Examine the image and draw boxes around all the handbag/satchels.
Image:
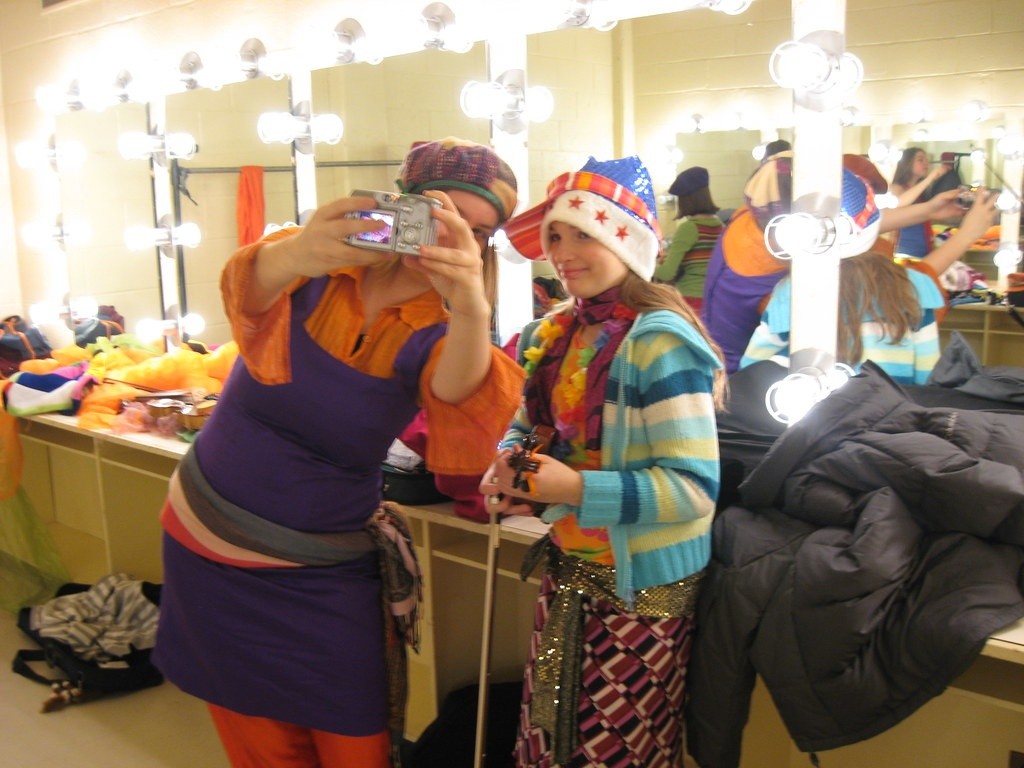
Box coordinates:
[12,581,165,714]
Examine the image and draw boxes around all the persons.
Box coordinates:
[479,153,727,768]
[156,134,526,768]
[652,141,1002,385]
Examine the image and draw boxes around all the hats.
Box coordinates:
[744,154,887,260]
[395,136,518,229]
[668,166,708,195]
[502,155,660,284]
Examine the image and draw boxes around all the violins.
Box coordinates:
[505,422,556,525]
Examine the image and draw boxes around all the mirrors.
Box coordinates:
[52,0,1023,372]
[676,114,1023,285]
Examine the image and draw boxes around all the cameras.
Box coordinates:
[344,189,444,254]
[954,183,1002,210]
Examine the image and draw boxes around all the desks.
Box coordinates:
[0,381,1024,768]
[938,302,1023,372]
